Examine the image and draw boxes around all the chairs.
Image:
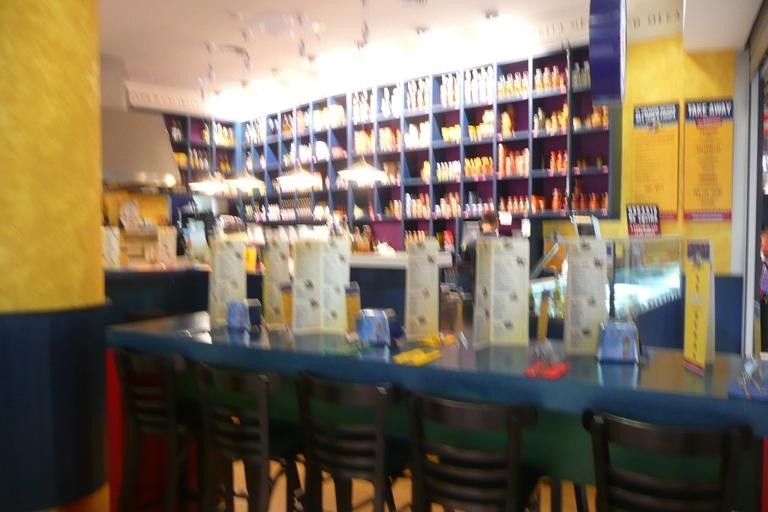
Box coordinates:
[117,345,753,511]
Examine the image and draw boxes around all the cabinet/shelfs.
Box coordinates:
[162,41,620,252]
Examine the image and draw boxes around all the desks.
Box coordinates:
[105,309,768,511]
